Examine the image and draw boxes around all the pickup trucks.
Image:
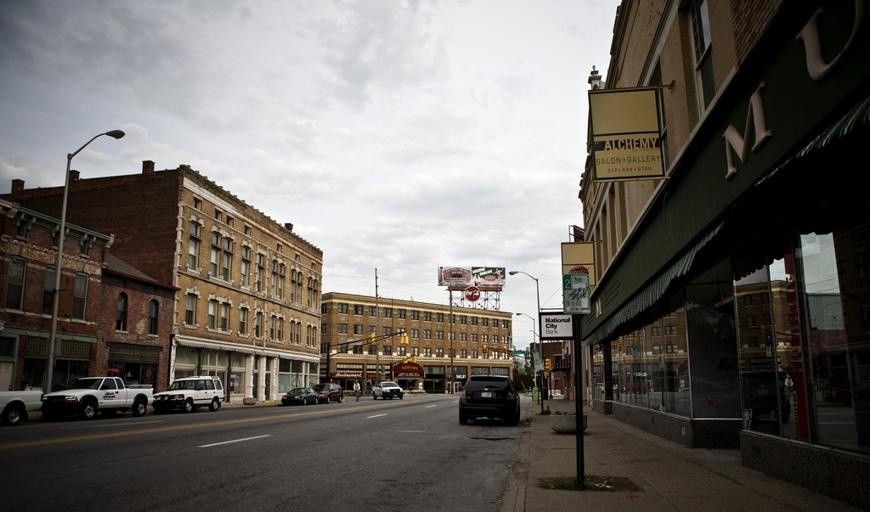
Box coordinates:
[0,376,155,425]
[372,381,403,400]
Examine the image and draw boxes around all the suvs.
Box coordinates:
[152,375,225,413]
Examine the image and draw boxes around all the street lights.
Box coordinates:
[509,271,543,413]
[46,130,125,394]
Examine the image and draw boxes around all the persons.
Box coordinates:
[353,379,360,396]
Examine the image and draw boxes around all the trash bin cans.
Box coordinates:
[532,387,539,400]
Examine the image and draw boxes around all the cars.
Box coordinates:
[459,374,519,427]
[281,383,343,406]
